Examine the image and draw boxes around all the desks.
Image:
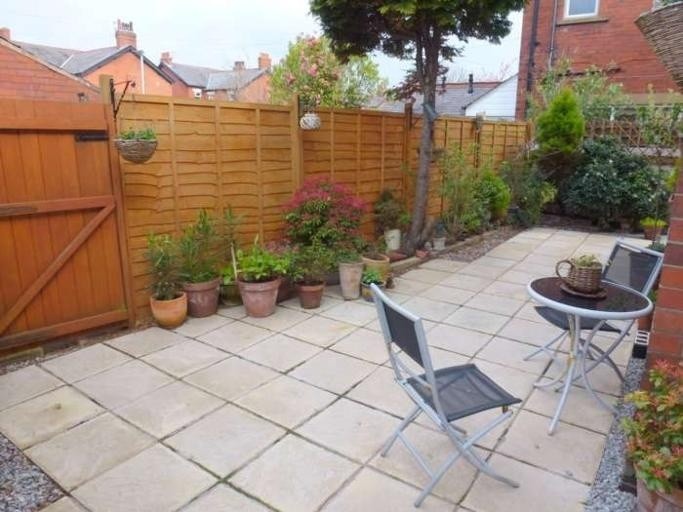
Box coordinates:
[523,272,652,435]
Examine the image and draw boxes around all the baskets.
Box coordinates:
[555,260,602,293]
[634,2,683,87]
[113,140,158,163]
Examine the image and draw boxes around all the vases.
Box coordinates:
[630,460,682,512]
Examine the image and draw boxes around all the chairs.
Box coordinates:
[366,281,524,507]
[523,238,663,392]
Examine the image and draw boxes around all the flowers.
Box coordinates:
[618,359,682,493]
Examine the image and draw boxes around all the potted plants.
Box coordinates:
[640,217,666,239]
[432,218,448,251]
[142,188,404,330]
[565,252,602,295]
[298,93,321,130]
[114,123,158,163]
